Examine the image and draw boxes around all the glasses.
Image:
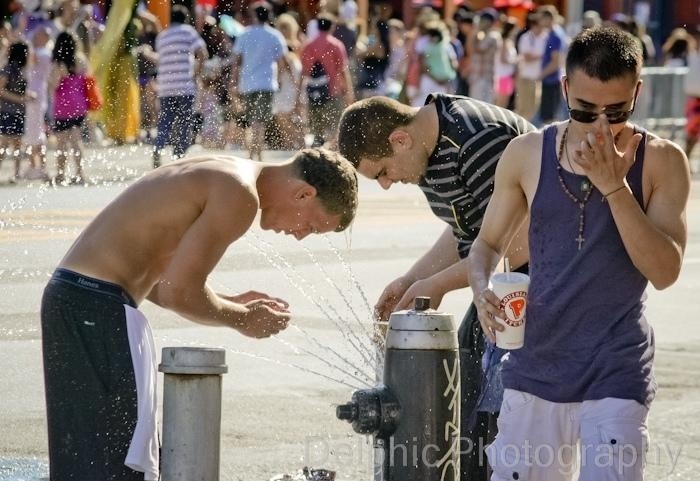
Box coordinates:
[564,81,640,125]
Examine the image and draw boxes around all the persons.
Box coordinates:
[469,27,692,479]
[0,0,699,191]
[38,146,360,480]
[335,93,545,480]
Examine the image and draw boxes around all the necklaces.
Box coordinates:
[556,119,626,252]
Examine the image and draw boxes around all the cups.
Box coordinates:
[492,269,529,351]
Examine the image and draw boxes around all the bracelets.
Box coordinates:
[601,183,628,199]
